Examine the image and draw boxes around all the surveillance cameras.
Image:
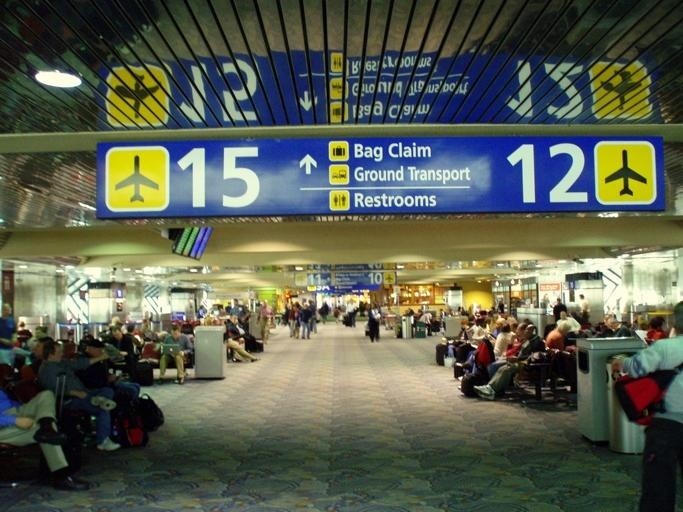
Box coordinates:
[573,259,583,264]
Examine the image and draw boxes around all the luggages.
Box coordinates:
[39,370,81,479]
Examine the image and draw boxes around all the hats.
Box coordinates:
[87,339,104,348]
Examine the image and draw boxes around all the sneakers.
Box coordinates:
[90,395,117,411]
[95,435,121,452]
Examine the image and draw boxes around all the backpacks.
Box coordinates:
[435,337,497,401]
[132,361,154,386]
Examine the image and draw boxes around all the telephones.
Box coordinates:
[575,335,644,454]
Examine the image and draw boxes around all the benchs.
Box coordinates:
[153,335,194,368]
[514,339,547,391]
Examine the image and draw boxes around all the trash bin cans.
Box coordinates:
[384,314,396,329]
[248,312,261,339]
[193,326,225,379]
[401,316,411,338]
[415,322,424,337]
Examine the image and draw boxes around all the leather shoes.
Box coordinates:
[54,474,89,490]
[32,428,67,446]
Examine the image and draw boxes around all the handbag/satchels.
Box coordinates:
[111,391,165,448]
[614,369,677,422]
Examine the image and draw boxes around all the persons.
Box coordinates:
[0,298,274,490]
[334,299,383,342]
[609,301,683,511]
[281,298,328,339]
[441,294,638,400]
[385,308,433,336]
[644,317,666,343]
